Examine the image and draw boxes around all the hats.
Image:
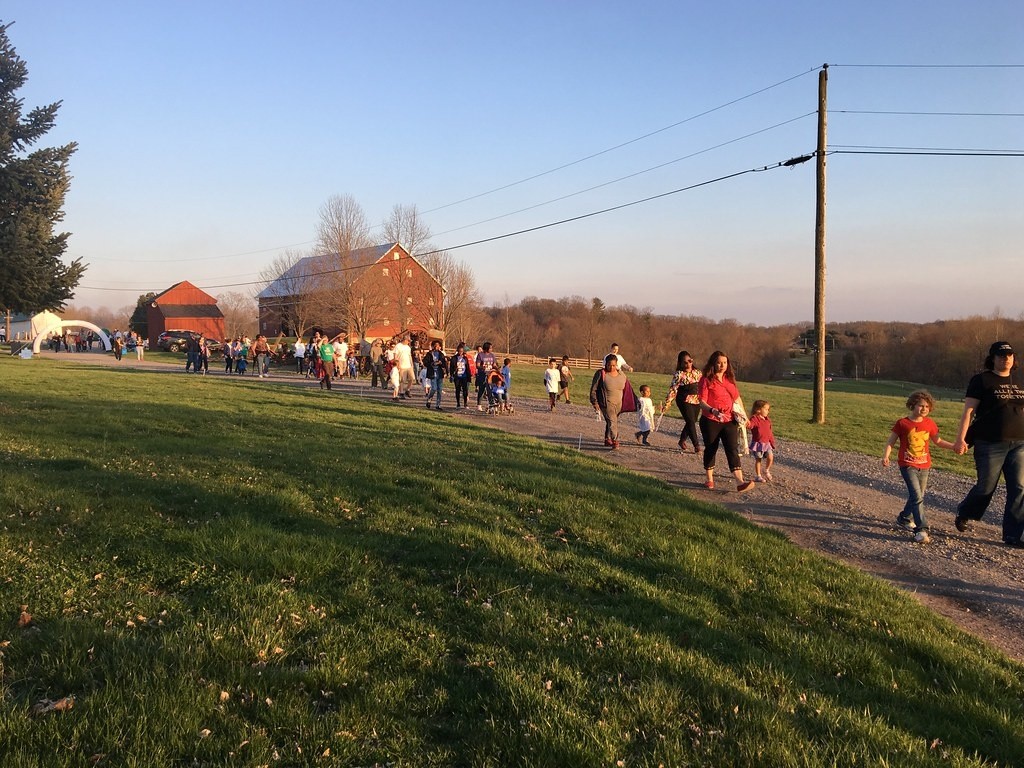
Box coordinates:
[990,341,1014,356]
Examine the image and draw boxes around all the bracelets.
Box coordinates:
[709,407,713,413]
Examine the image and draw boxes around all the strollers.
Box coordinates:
[484,365,515,417]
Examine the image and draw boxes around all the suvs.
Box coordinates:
[157,330,220,353]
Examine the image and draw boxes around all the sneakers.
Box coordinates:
[1004,538,1024,549]
[955,503,968,532]
[915,531,929,543]
[897,516,916,532]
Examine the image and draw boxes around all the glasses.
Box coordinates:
[683,359,693,364]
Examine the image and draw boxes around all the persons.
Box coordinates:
[47,330,62,353]
[455,342,466,354]
[476,342,500,412]
[185,332,275,378]
[422,340,446,410]
[882,390,965,544]
[662,350,702,455]
[635,385,661,446]
[420,364,431,398]
[696,351,754,492]
[590,354,641,450]
[743,399,776,483]
[473,346,488,399]
[603,343,633,373]
[439,342,450,378]
[370,338,423,401]
[110,329,143,362]
[450,346,472,410]
[294,331,357,390]
[557,355,574,404]
[543,358,561,413]
[0,326,6,343]
[61,329,93,353]
[492,380,510,412]
[502,358,511,387]
[952,342,1024,549]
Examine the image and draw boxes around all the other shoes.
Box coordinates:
[678,441,687,449]
[695,446,702,453]
[635,433,640,443]
[556,395,560,400]
[565,400,571,404]
[477,404,482,411]
[705,480,713,489]
[755,476,766,483]
[737,480,754,493]
[259,371,470,412]
[604,439,614,446]
[763,468,772,480]
[642,440,650,446]
[612,440,620,450]
